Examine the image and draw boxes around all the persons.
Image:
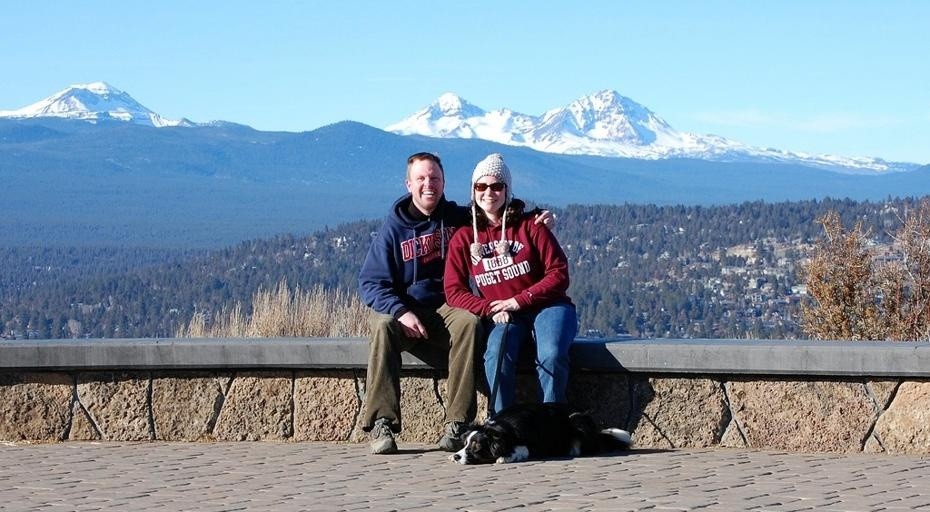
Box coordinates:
[358,153,555,454]
[443,154,578,415]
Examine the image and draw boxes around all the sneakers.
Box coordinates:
[439,422,464,452]
[368,418,398,454]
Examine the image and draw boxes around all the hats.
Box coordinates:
[470,154,511,205]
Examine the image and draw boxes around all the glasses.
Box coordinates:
[475,182,505,191]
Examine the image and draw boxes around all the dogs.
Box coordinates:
[448,401,633,465]
